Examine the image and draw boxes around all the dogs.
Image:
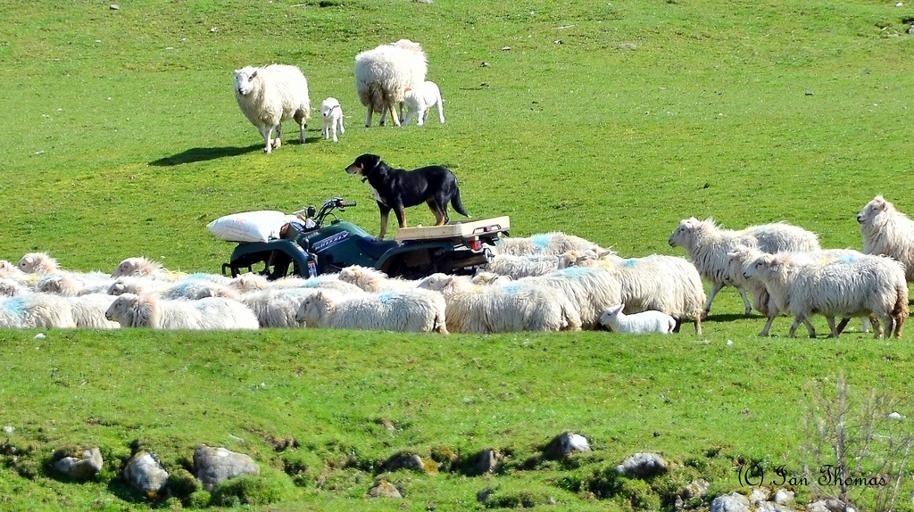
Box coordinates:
[345,154,469,241]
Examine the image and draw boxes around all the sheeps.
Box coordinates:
[234,61,310,154]
[321,97,345,143]
[856,192,914,282]
[669,217,909,342]
[0,230,708,341]
[400,81,445,127]
[354,38,427,127]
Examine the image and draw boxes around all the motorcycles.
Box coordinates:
[204,195,513,282]
